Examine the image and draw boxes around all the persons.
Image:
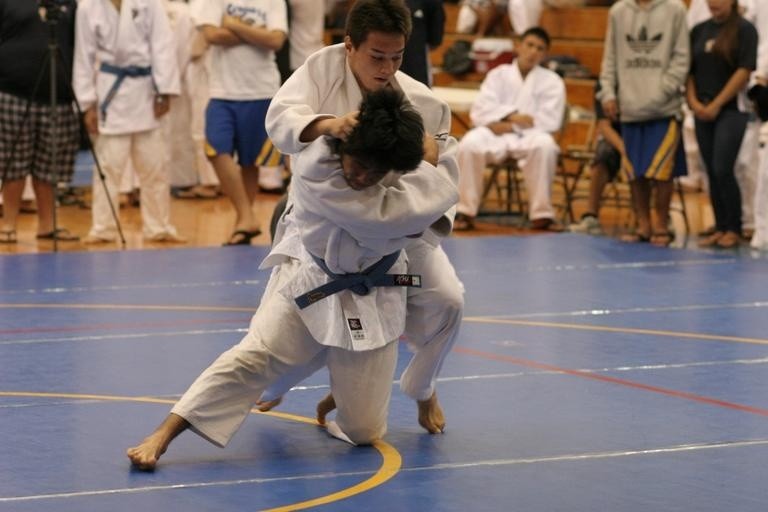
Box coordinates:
[258,0,467,437]
[0,0,344,246]
[399,0,767,263]
[122,90,458,471]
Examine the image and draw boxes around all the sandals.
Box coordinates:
[618,229,650,244]
[143,230,187,244]
[648,230,675,249]
[696,226,742,251]
[0,180,287,219]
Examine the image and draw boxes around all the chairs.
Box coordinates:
[471,103,690,237]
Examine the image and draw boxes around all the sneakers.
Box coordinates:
[565,215,603,235]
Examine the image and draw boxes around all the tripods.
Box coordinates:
[1,42,127,251]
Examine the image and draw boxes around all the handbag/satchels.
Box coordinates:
[442,39,476,78]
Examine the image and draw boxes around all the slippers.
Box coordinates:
[451,212,475,232]
[36,227,80,241]
[170,238,171,239]
[531,219,566,232]
[80,234,117,246]
[0,226,17,243]
[221,230,262,246]
